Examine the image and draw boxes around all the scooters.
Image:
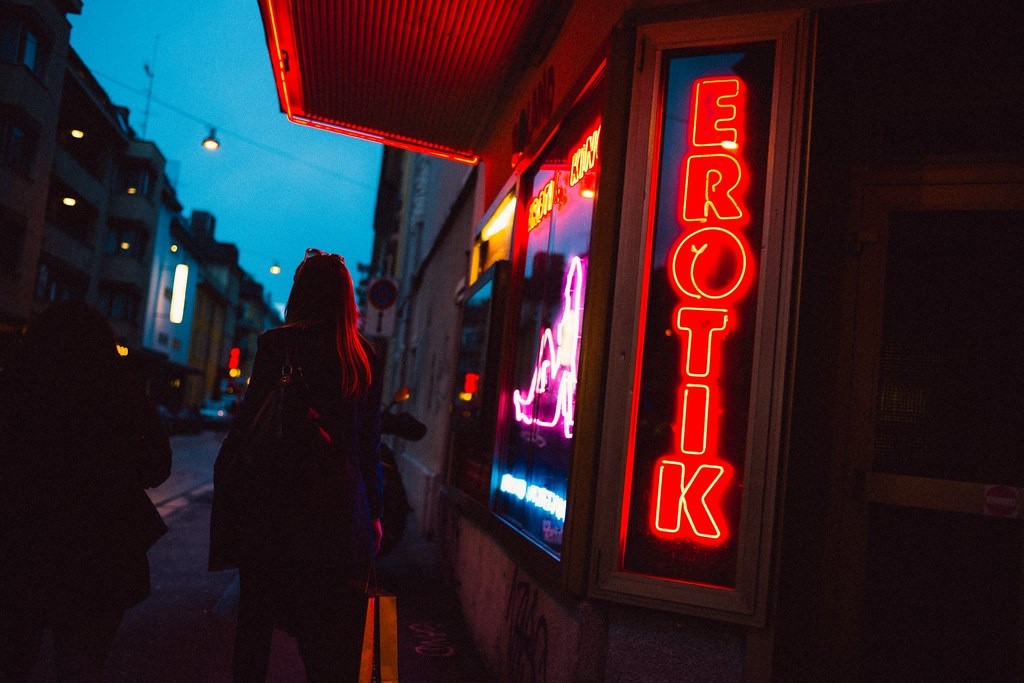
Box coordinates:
[375,385,429,561]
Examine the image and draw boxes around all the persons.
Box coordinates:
[0,304,171,683]
[209,248,383,683]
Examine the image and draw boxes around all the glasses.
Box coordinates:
[305,248,345,265]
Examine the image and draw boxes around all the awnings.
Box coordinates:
[257,0,539,164]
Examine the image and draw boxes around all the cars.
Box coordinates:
[153,394,239,433]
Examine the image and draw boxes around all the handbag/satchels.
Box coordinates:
[207,326,373,577]
